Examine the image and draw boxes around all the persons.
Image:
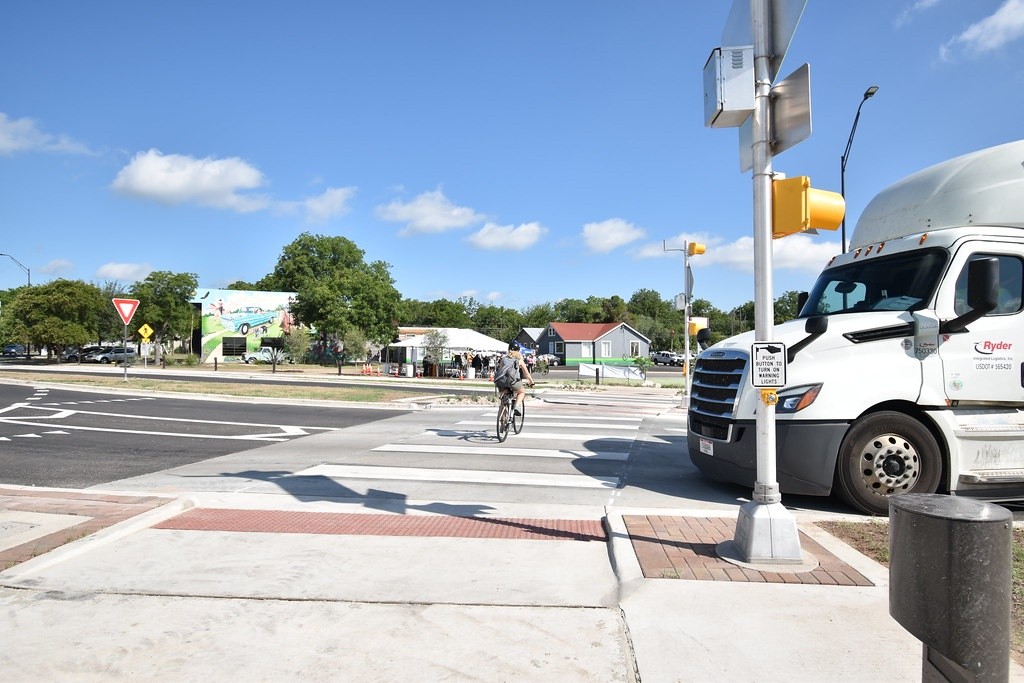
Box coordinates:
[494,342,534,431]
[367,350,549,379]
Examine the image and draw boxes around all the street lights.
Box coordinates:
[0,252,32,361]
[839,85,882,311]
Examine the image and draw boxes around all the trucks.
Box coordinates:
[683,137,1024,517]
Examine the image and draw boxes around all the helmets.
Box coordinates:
[508,342,522,349]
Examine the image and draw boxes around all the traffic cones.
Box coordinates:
[458,369,465,380]
[376,367,381,377]
[416,369,422,378]
[488,371,494,381]
[394,367,400,377]
[362,363,374,376]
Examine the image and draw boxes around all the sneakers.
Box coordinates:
[514,405,522,416]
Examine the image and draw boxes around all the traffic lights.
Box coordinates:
[772,174,847,242]
[689,322,706,336]
[687,241,707,257]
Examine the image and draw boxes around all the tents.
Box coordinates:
[389,328,511,376]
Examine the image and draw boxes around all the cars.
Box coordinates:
[64,344,137,364]
[535,353,562,367]
[649,349,697,366]
[2,344,24,357]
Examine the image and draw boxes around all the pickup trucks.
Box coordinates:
[241,347,293,365]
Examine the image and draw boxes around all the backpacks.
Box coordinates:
[494,356,519,389]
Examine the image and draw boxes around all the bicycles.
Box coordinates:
[496,381,536,443]
